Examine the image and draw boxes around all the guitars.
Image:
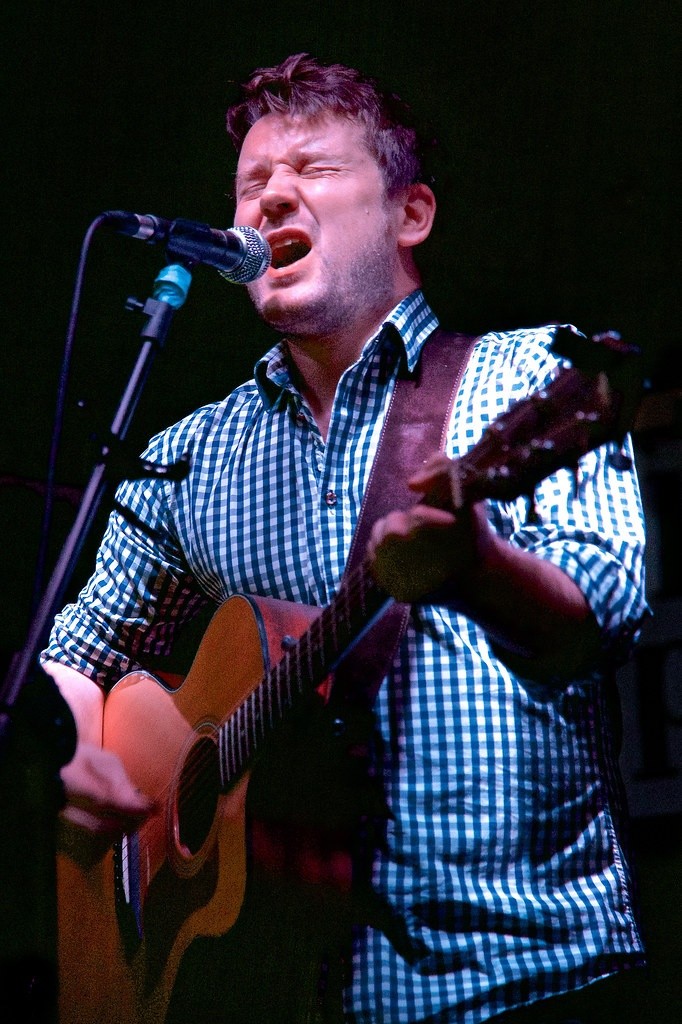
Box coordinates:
[57,325,645,1024]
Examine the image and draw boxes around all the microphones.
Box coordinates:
[101,210,272,284]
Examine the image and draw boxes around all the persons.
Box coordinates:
[39,50,659,1024]
